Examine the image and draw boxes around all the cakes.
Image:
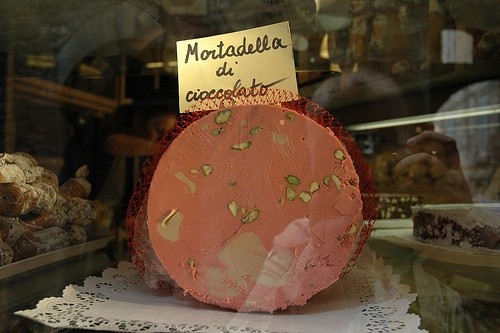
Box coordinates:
[411,202,500,255]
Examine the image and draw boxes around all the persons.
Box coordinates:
[56,88,178,257]
[224,69,499,333]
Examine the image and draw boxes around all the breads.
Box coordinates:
[1,151,116,267]
